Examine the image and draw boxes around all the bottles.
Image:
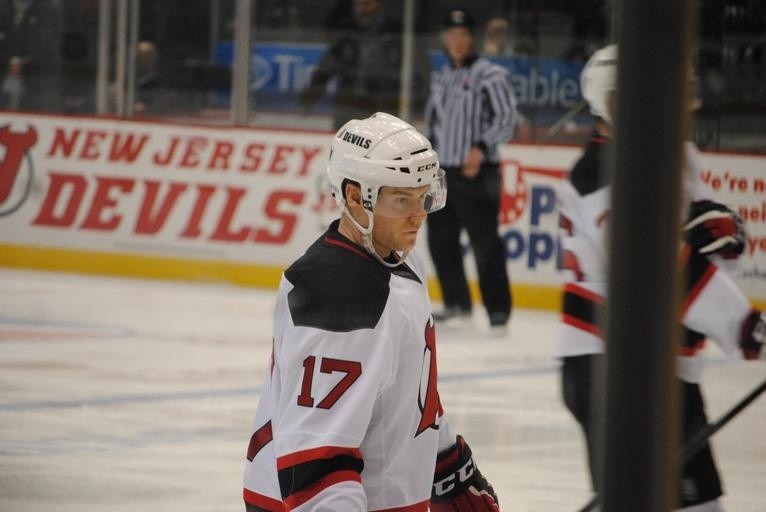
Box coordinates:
[439,9,478,28]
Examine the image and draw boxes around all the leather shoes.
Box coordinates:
[684,199,747,261]
[429,430,501,512]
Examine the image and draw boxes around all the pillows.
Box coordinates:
[578,42,619,124]
[325,112,441,213]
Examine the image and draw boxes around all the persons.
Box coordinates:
[2,3,229,122]
[423,10,521,327]
[242,110,500,509]
[303,1,429,137]
[549,45,766,510]
[556,2,764,148]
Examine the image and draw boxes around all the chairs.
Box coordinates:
[432,305,508,325]
